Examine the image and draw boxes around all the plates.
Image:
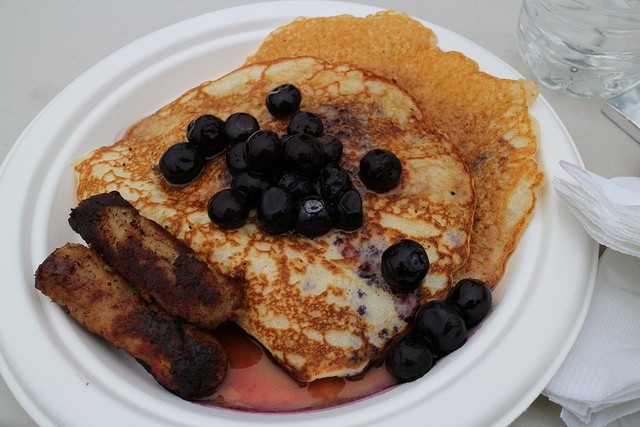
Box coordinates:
[1,0,600,427]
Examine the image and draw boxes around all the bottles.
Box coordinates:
[519,2,639,101]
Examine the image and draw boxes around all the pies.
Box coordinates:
[73,8,539,391]
